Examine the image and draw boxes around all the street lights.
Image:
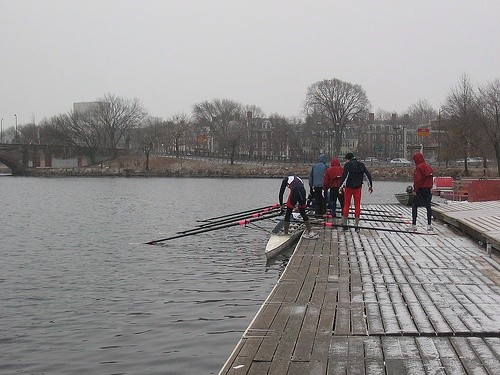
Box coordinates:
[1,118,3,143]
[14,114,17,143]
[438,108,453,162]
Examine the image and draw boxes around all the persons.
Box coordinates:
[323,159,344,218]
[279,175,314,237]
[309,154,327,214]
[339,153,373,231]
[409,153,434,231]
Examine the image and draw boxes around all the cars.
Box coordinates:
[362,157,379,163]
[457,157,490,163]
[390,158,412,167]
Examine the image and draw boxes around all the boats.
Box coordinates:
[265,210,307,260]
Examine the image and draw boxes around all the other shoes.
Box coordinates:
[412,225,418,231]
[303,232,320,239]
[427,225,433,230]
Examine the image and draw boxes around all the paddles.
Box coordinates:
[143,203,438,245]
